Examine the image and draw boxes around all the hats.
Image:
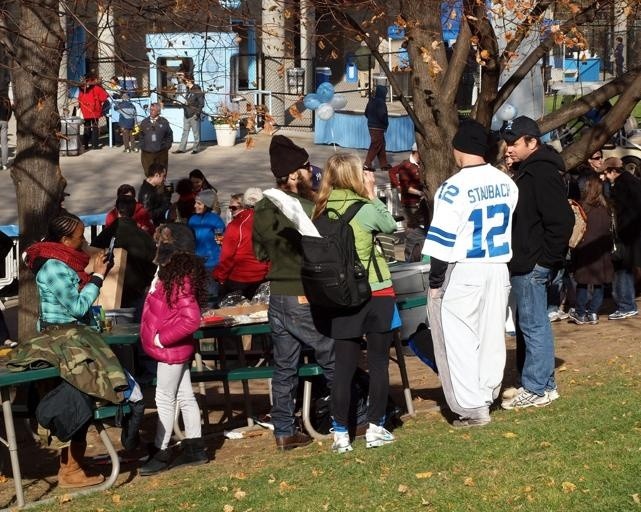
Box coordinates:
[243,187,263,206]
[411,142,417,151]
[269,134,309,176]
[195,189,215,208]
[452,119,485,157]
[598,157,623,170]
[503,115,540,136]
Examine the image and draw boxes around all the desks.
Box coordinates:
[101,292,233,435]
[234,294,430,415]
[314,109,414,153]
[0,362,60,512]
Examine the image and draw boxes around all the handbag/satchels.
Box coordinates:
[567,192,588,251]
[610,206,626,262]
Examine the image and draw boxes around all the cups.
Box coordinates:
[214,228,223,241]
[101,317,115,334]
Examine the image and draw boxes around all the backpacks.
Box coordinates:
[299,200,384,312]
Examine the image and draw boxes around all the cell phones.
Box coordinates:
[107,236,116,261]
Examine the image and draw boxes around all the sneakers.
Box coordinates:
[452,418,490,427]
[123,149,130,153]
[548,389,560,400]
[558,310,569,319]
[501,391,551,410]
[381,164,392,171]
[365,426,395,448]
[568,311,585,324]
[608,309,638,320]
[132,148,139,152]
[585,313,599,324]
[363,167,376,172]
[332,432,353,454]
[548,312,561,322]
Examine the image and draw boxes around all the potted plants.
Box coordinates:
[210,106,240,147]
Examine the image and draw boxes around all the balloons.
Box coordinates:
[303,93,321,109]
[317,82,334,102]
[317,103,334,119]
[330,95,347,110]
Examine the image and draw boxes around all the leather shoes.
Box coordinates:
[354,425,368,440]
[276,433,311,451]
[191,150,197,154]
[171,150,183,154]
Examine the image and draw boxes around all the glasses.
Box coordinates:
[300,161,310,169]
[228,206,237,211]
[590,156,602,160]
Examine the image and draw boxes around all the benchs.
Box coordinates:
[148,362,323,439]
[619,116,641,151]
[1,399,131,491]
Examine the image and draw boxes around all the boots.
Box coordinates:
[139,444,179,476]
[57,442,104,488]
[168,438,208,470]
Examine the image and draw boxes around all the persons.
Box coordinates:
[173,75,204,154]
[614,37,624,76]
[599,157,641,320]
[89,196,153,274]
[211,187,271,308]
[587,150,603,170]
[499,140,518,335]
[106,76,123,94]
[568,171,615,324]
[105,185,151,231]
[399,142,424,217]
[136,103,173,176]
[389,159,408,192]
[421,119,520,429]
[134,222,209,476]
[249,135,369,453]
[189,190,225,267]
[216,193,244,245]
[137,163,178,221]
[167,179,195,220]
[301,147,397,454]
[548,172,586,321]
[189,169,221,215]
[23,214,115,489]
[501,115,576,412]
[77,75,108,149]
[113,91,140,154]
[360,85,395,172]
[300,147,324,191]
